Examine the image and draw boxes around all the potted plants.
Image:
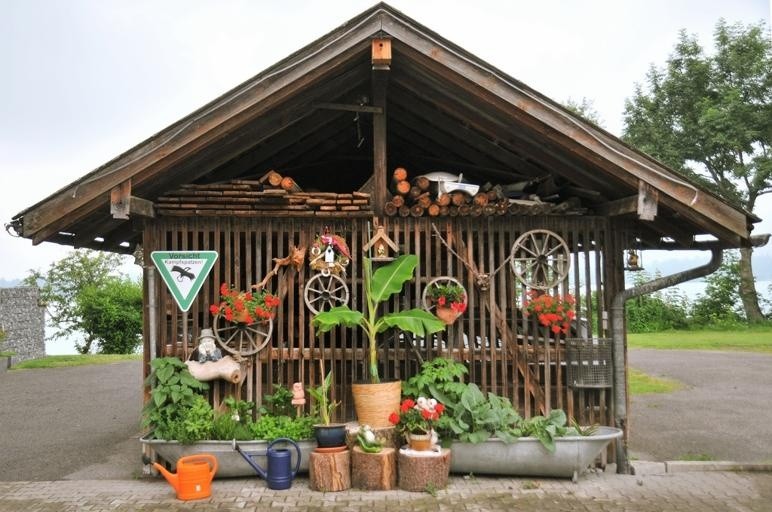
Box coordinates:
[399,354,631,483]
[307,370,355,451]
[137,354,321,479]
[310,253,447,428]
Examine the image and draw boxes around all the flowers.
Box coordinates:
[388,392,445,434]
[517,289,578,334]
[427,282,468,315]
[209,281,283,327]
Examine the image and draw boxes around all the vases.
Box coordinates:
[235,307,250,322]
[407,430,432,451]
[436,306,458,325]
